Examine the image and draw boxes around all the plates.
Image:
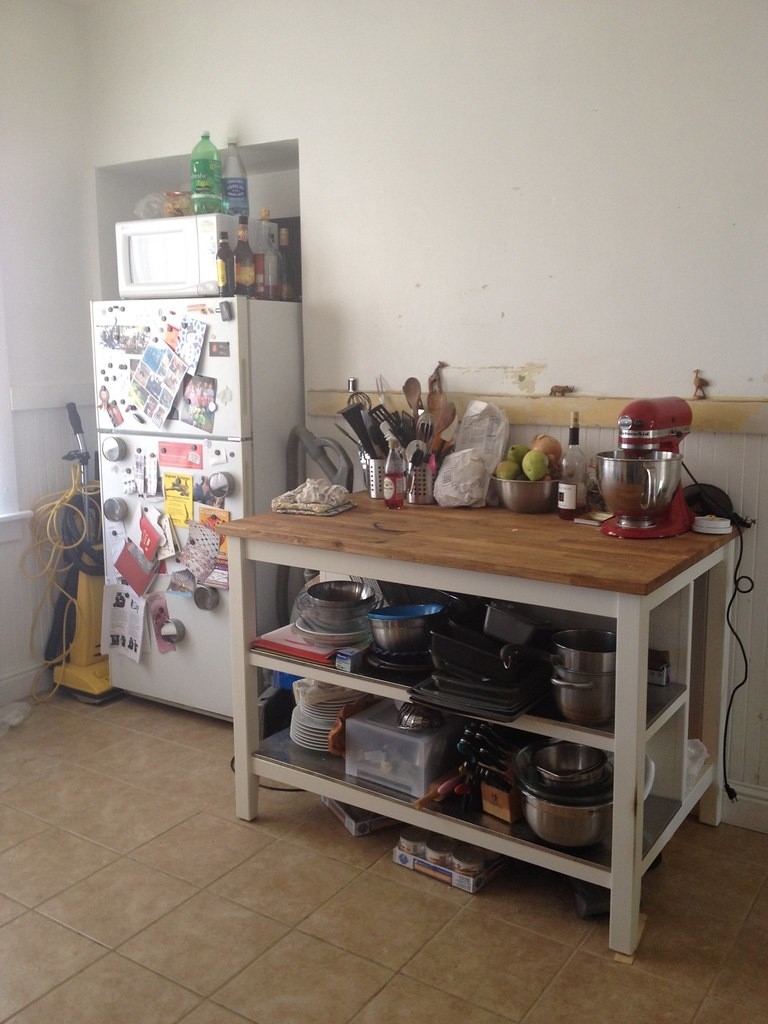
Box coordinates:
[298,689,369,721]
[289,705,334,752]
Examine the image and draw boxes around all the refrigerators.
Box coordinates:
[90,293,306,723]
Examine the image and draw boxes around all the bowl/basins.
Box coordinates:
[596,450,683,518]
[367,604,444,651]
[491,473,558,513]
[397,702,443,730]
[291,581,383,648]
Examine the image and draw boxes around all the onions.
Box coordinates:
[531,432,561,468]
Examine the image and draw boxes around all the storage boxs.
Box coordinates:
[391,839,511,894]
[320,795,403,837]
[344,698,473,800]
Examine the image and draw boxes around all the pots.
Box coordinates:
[513,740,613,847]
[549,627,616,726]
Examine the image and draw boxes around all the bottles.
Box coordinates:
[383,440,406,510]
[254,209,279,300]
[558,411,587,520]
[279,228,297,301]
[222,136,249,216]
[216,232,234,297]
[190,131,223,214]
[233,216,254,297]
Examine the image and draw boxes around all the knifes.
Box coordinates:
[459,723,512,772]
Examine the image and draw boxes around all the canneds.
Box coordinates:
[399,827,503,877]
[162,191,193,217]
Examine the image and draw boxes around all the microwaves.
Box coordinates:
[115,212,279,300]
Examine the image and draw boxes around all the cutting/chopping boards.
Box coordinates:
[214,490,746,596]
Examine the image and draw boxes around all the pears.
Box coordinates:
[496,443,549,482]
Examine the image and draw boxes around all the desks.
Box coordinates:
[214,486,746,967]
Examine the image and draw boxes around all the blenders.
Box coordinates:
[596,396,699,539]
